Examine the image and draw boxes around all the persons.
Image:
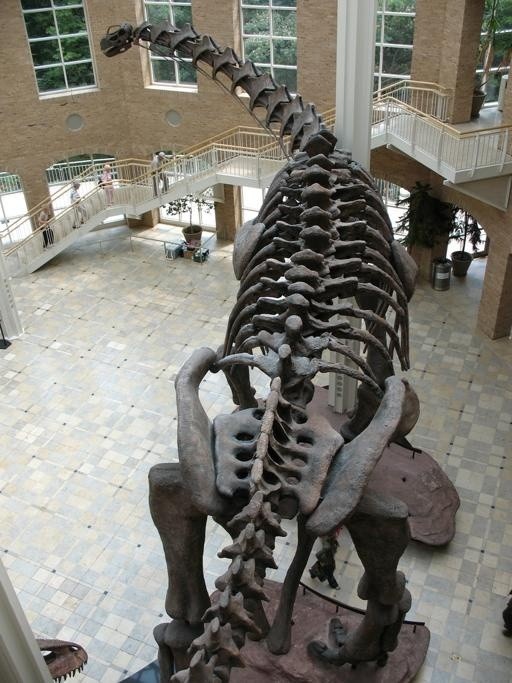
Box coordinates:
[70,182,86,229]
[150,150,169,198]
[100,164,114,207]
[36,203,54,249]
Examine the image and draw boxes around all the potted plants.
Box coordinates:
[470,0,511,121]
[162,184,216,243]
[394,181,490,277]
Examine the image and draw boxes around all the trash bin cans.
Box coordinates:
[431,257,451,290]
